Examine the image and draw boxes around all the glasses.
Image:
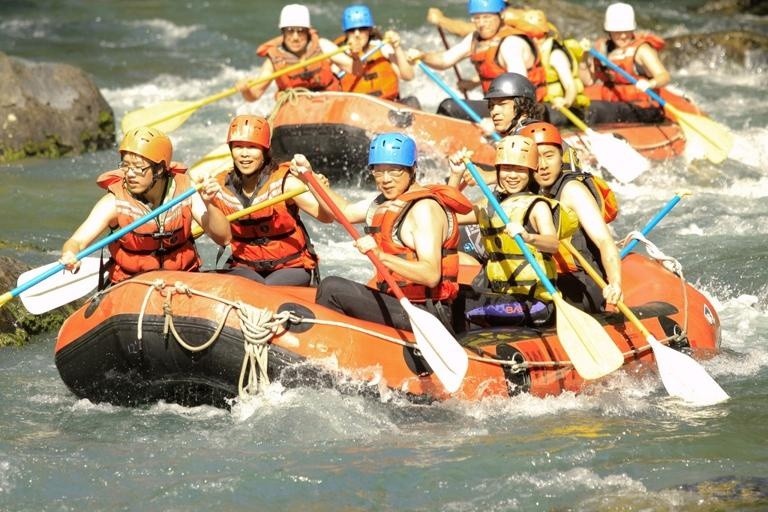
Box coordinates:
[368,167,408,177]
[117,159,160,174]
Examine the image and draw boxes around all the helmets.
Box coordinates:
[116,126,175,170]
[225,112,272,151]
[488,134,540,174]
[519,120,564,152]
[341,4,374,33]
[467,0,505,17]
[602,2,637,33]
[364,131,418,171]
[277,4,313,34]
[482,71,536,103]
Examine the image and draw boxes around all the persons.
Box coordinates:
[444,135,560,331]
[235,1,365,101]
[336,3,416,107]
[471,123,629,316]
[58,125,231,292]
[207,113,330,289]
[406,2,547,122]
[479,65,581,176]
[575,1,672,126]
[505,7,590,127]
[288,134,470,337]
[424,0,519,37]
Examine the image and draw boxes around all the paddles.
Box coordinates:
[292,158,468,394]
[462,157,625,380]
[0,181,209,307]
[589,48,731,165]
[16,182,311,316]
[549,94,651,184]
[560,238,731,408]
[122,41,353,134]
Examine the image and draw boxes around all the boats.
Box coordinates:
[54,250,724,413]
[263,84,712,189]
